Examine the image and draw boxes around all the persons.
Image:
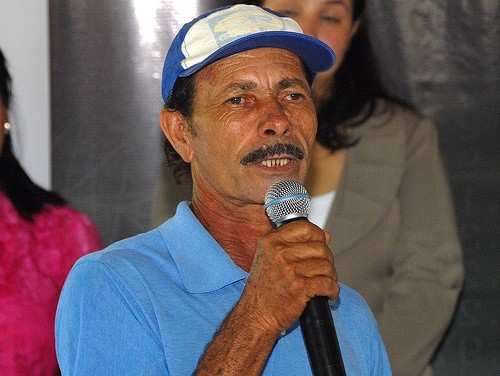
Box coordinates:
[0,50,109,376]
[53,3,393,376]
[216,0,464,375]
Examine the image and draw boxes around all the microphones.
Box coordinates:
[264,180,347,376]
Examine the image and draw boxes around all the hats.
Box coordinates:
[161,3,337,104]
